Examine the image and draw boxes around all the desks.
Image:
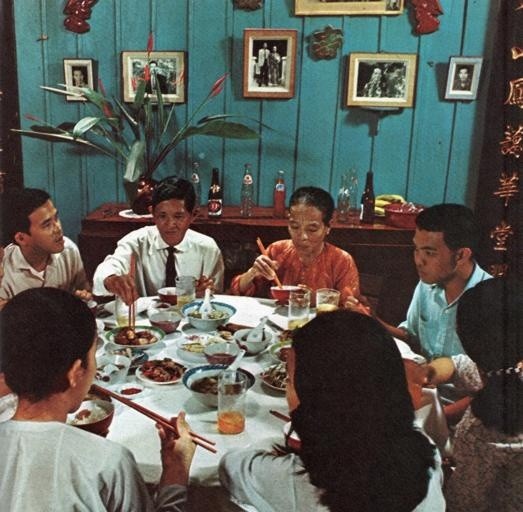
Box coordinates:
[79,201,430,327]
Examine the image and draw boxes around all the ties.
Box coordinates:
[165,246,175,287]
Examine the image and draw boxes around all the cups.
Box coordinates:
[218,370,247,434]
[175,277,196,311]
[287,288,310,330]
[316,288,341,313]
[115,295,136,328]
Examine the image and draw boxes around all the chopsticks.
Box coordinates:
[128,256,136,328]
[255,236,283,290]
[345,287,371,318]
[269,410,290,423]
[91,383,218,454]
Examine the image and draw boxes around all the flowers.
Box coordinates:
[8,24,285,186]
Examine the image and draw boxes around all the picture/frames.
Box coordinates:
[293,0,406,21]
[119,49,191,106]
[442,54,485,104]
[60,58,95,106]
[241,28,298,100]
[344,50,420,112]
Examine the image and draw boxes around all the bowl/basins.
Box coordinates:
[64,400,114,436]
[182,365,254,409]
[384,201,425,228]
[269,285,302,305]
[86,301,97,320]
[157,286,177,305]
[148,311,180,333]
[94,355,131,387]
[104,326,165,349]
[180,301,236,332]
[282,422,301,451]
[232,328,271,355]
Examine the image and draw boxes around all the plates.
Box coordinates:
[118,384,144,399]
[135,360,186,384]
[269,341,292,364]
[259,363,290,391]
[177,335,222,357]
[120,351,147,370]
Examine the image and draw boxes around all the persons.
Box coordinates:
[362,62,386,99]
[71,68,86,91]
[217,309,447,512]
[454,66,473,92]
[229,185,360,306]
[257,40,270,87]
[403,270,523,510]
[267,46,284,88]
[345,203,498,361]
[92,175,224,307]
[142,60,167,95]
[0,287,197,510]
[2,187,92,303]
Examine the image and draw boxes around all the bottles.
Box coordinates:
[336,166,374,224]
[241,162,255,218]
[208,168,222,217]
[272,170,286,218]
[190,161,202,210]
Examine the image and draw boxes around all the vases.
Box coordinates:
[124,176,160,217]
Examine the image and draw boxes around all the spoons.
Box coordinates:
[199,288,213,313]
[221,349,246,385]
[246,316,268,342]
